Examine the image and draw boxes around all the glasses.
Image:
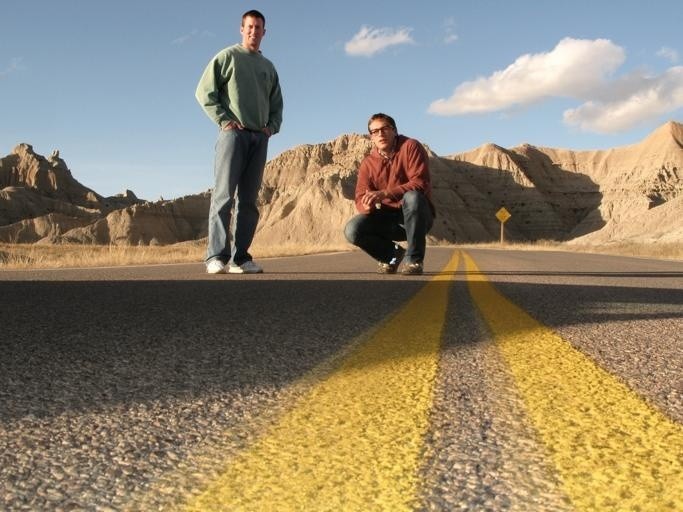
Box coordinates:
[369,125,395,135]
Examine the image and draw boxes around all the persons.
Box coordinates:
[192,9,284,275]
[343,112,436,275]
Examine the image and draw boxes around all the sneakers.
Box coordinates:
[203,259,226,275]
[375,244,407,275]
[399,261,424,275]
[228,260,265,273]
